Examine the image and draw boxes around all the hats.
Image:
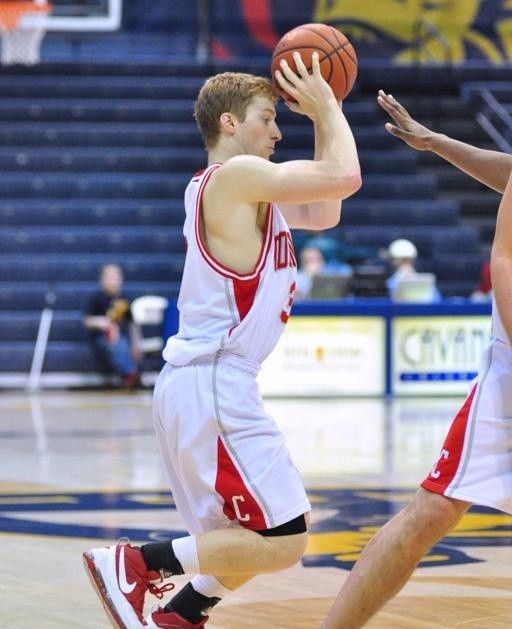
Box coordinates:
[388,238,417,259]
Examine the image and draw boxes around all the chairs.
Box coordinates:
[0,1,491,389]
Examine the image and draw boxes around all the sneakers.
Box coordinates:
[143,605,211,629]
[82,535,175,629]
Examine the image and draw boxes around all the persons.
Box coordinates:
[378,237,441,304]
[73,42,364,629]
[318,85,510,629]
[285,244,355,300]
[80,262,147,389]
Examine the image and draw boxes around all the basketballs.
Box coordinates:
[271,24,358,106]
